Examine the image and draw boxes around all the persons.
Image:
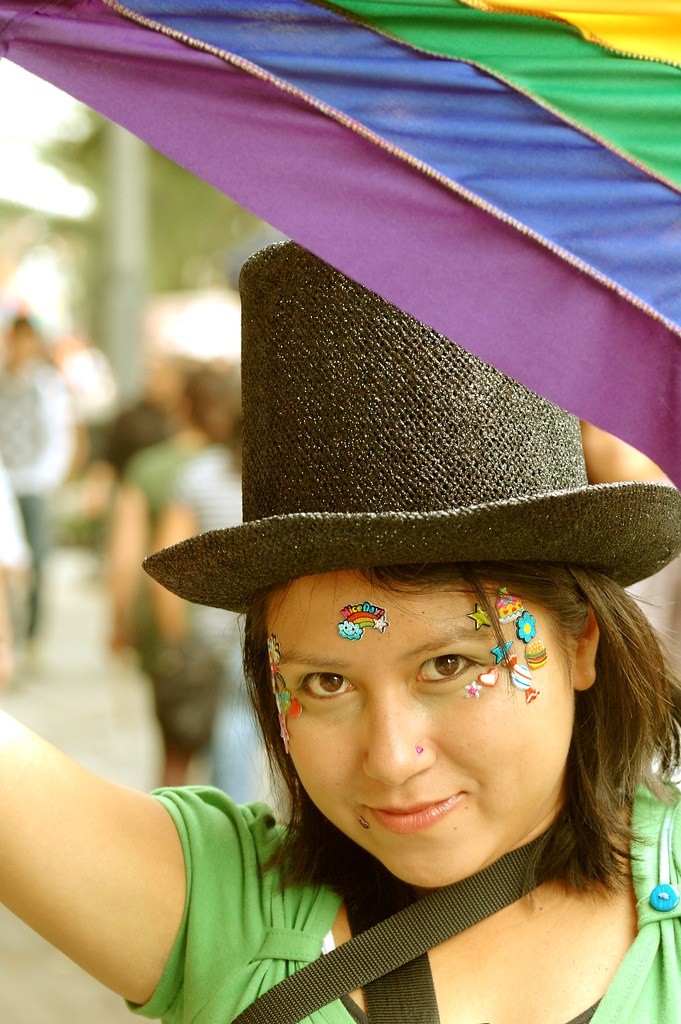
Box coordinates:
[0,241,680,1024]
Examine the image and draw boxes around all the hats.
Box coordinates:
[143,238,681,614]
